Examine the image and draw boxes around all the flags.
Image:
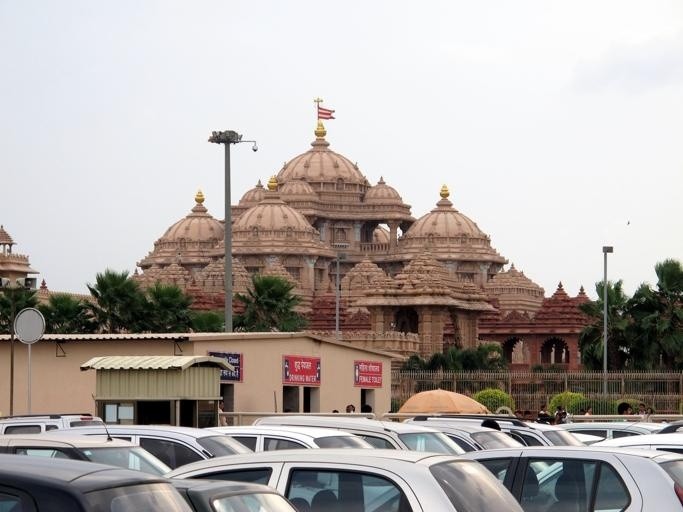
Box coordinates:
[318,107,335,119]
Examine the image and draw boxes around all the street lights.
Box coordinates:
[600,246,612,402]
[207,130,260,331]
[335,252,346,340]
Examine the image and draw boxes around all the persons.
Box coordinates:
[514,404,568,425]
[333,405,374,413]
[617,402,653,415]
[585,407,592,415]
[219,400,228,426]
